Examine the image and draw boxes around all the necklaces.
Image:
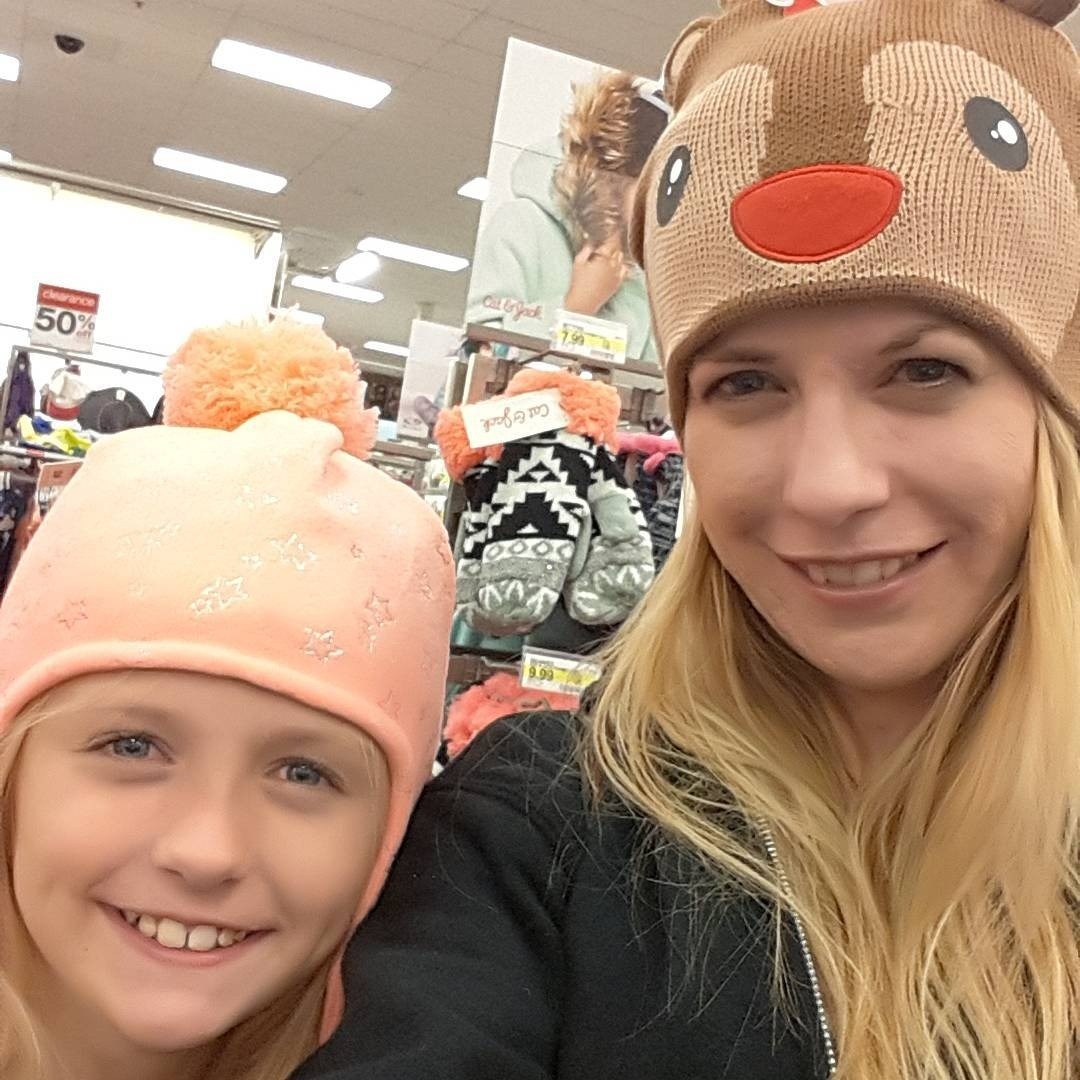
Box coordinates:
[750,801,843,1080]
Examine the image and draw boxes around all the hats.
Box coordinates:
[623,0,1079,422]
[3,308,457,1043]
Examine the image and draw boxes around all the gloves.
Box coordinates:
[434,368,656,637]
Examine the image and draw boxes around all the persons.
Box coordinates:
[465,97,665,374]
[1,420,459,1080]
[279,49,1078,1080]
